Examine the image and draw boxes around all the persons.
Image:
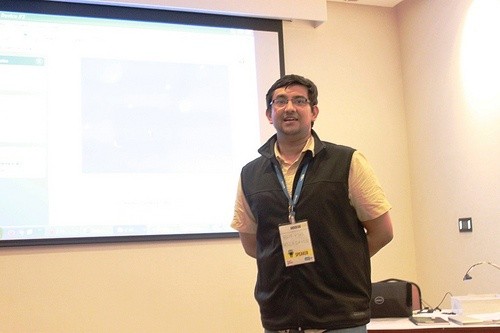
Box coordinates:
[231,73,395,333]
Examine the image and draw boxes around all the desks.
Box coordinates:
[366,309,500,333]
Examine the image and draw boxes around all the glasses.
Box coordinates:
[269,97,310,107]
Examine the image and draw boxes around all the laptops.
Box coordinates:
[370,281,412,319]
